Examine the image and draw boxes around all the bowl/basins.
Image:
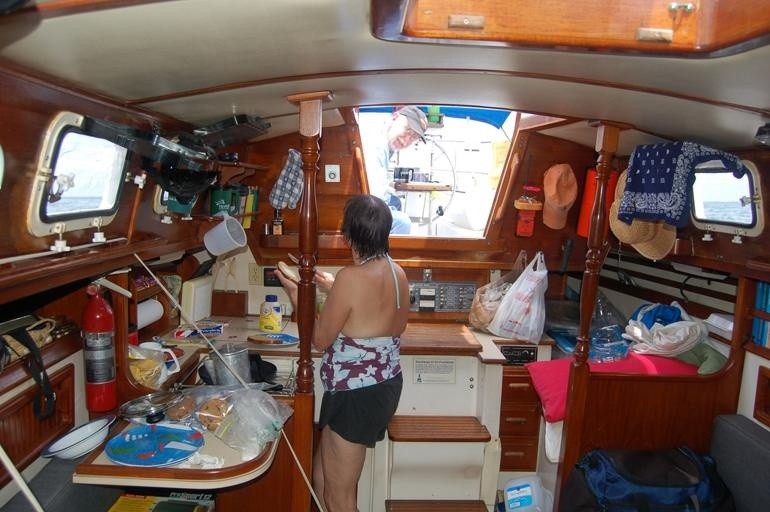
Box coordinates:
[39,413,114,461]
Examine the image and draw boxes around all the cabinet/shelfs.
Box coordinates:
[498,374,543,474]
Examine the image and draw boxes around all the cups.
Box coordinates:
[204,210,248,257]
[139,342,180,385]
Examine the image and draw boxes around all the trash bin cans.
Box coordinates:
[558,446,735,512]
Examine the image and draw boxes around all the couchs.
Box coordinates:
[559,413,769,512]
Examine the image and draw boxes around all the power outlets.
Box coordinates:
[247,262,262,285]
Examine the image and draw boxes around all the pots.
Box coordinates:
[199,361,283,390]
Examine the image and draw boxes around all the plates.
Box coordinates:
[165,347,185,363]
[104,424,205,469]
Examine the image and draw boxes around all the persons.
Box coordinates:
[360,106,428,234]
[273,196,409,512]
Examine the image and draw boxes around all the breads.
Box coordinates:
[247,336,283,343]
[277,261,299,286]
[166,396,196,420]
[200,398,233,430]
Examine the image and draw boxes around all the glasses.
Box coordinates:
[401,121,420,142]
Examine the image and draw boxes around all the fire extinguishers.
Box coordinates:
[83,275,132,412]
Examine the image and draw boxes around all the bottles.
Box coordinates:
[270,207,284,236]
[259,295,283,335]
[589,294,609,350]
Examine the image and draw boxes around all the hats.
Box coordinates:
[396,105,430,143]
[609,169,677,260]
[542,162,579,230]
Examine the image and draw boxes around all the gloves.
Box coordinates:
[268,148,305,210]
